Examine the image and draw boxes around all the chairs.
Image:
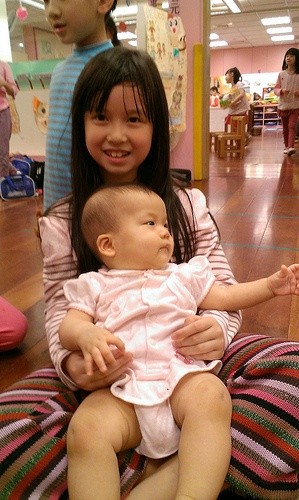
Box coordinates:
[210,116,246,159]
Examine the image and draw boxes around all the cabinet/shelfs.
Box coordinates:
[253,104,281,125]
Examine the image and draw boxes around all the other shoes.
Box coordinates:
[287,147,296,156]
[245,134,252,145]
[284,147,288,153]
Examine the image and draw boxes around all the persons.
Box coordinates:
[58,183,299,500]
[0,48,299,500]
[43,0,126,211]
[0,297,28,352]
[210,48,299,157]
[0,61,21,181]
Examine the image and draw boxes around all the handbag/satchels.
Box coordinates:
[0,153,45,200]
